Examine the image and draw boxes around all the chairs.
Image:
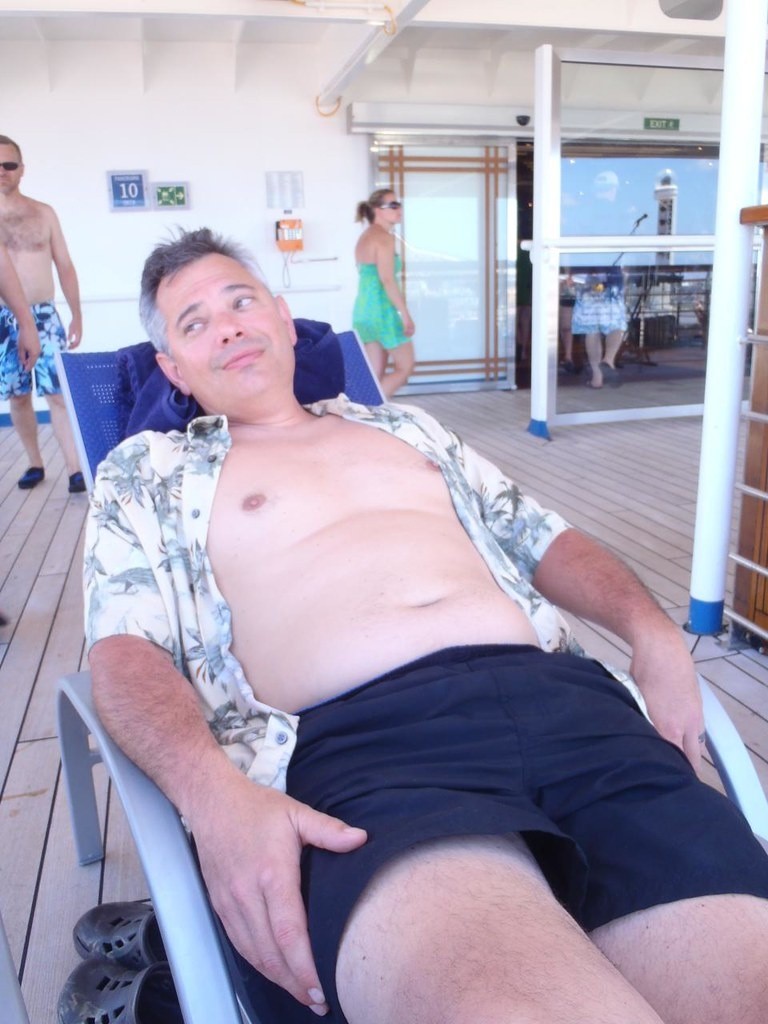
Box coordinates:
[50,329,768,1023]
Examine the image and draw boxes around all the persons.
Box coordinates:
[0,135,86,494]
[82,231,768,1024]
[351,189,415,400]
[517,189,629,389]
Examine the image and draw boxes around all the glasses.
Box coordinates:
[0,162,17,170]
[374,202,400,209]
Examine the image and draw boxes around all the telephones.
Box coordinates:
[275,218,304,251]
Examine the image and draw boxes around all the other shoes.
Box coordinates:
[19,467,44,489]
[68,471,86,492]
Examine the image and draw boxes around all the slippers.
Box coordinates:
[73,898,164,971]
[56,958,183,1024]
